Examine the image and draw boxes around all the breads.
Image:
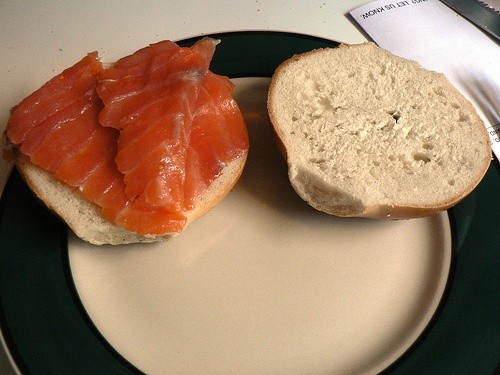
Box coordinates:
[9,148,249,246]
[267,42,492,217]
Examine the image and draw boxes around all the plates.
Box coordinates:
[67,78,452,375]
